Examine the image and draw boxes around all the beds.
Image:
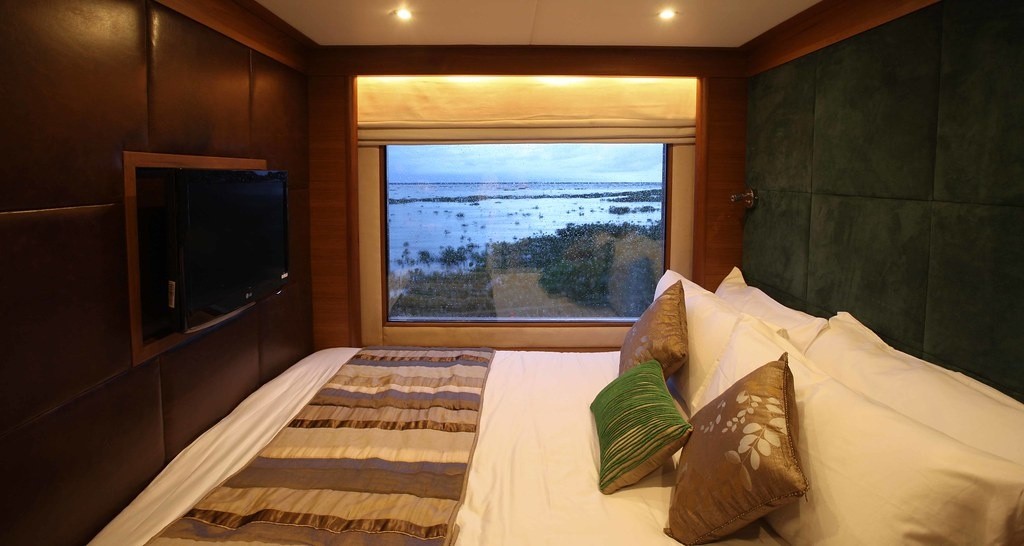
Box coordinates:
[88,347,789,546]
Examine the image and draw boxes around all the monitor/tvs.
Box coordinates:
[176,168,289,332]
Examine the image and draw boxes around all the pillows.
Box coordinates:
[714,266,827,355]
[619,280,688,380]
[589,360,694,495]
[665,352,810,546]
[654,268,790,415]
[689,314,1024,546]
[804,312,1024,466]
